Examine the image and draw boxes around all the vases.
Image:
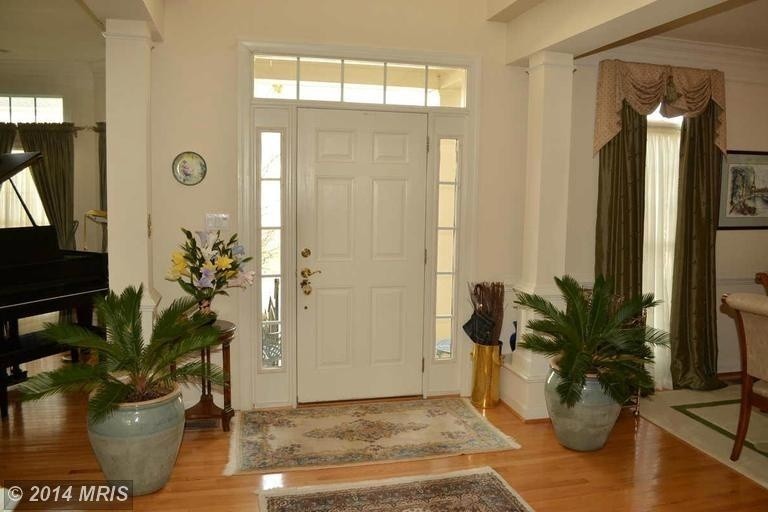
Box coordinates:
[198,298,211,314]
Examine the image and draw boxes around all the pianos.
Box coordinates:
[0,152,108,386]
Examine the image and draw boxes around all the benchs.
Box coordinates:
[0,322,107,417]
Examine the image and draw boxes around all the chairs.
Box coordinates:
[721,292,767,462]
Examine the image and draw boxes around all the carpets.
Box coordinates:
[627,381,767,490]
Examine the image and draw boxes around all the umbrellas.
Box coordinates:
[462,283,496,347]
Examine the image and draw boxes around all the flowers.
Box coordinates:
[164,227,256,298]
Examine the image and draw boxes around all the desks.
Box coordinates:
[169,319,237,432]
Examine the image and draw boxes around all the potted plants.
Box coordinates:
[512,273,673,451]
[16,281,232,497]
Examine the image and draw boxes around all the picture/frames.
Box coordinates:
[716,150,768,230]
